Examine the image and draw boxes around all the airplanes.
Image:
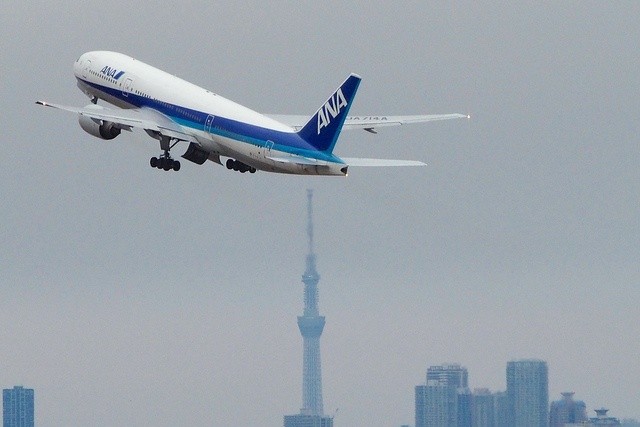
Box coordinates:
[34,51,472,176]
